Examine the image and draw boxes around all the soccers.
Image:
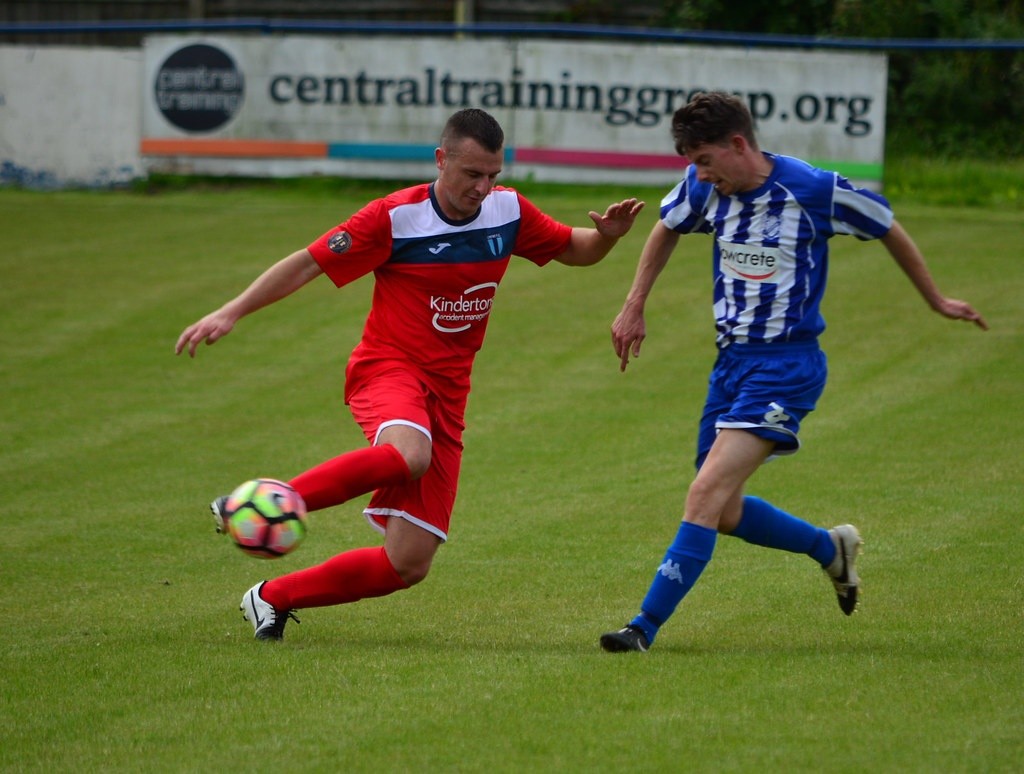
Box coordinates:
[224,477,309,561]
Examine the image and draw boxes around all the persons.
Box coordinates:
[174,109,644,643]
[600,92,988,654]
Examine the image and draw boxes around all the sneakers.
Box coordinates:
[210,495,232,536]
[823,524,861,616]
[599,626,649,655]
[240,578,302,646]
